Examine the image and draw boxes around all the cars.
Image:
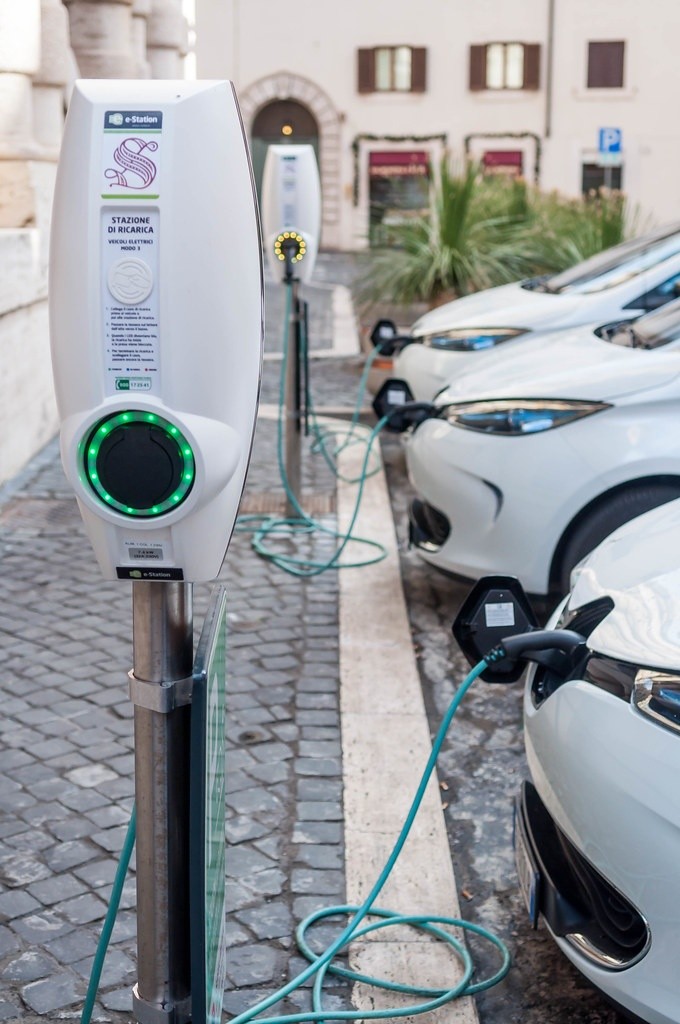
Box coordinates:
[508,498,679,1023]
[380,214,680,606]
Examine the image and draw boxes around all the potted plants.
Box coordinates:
[344,160,514,352]
